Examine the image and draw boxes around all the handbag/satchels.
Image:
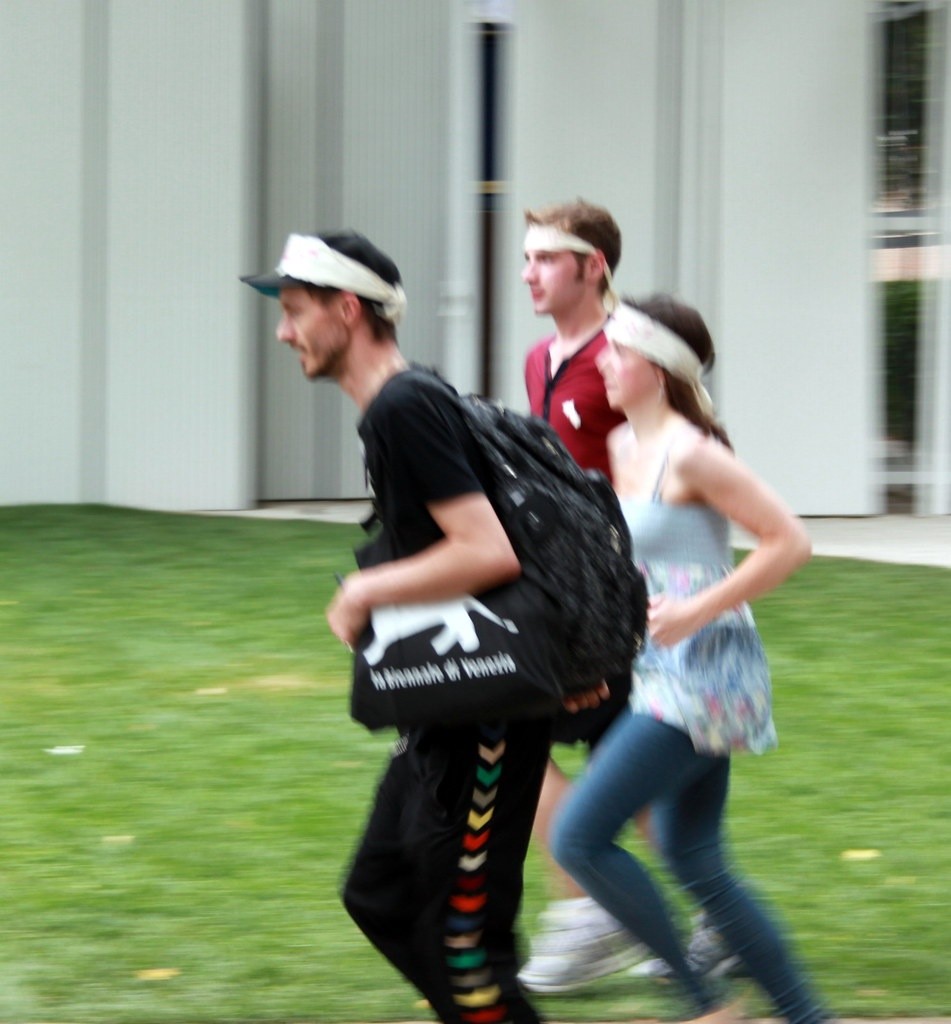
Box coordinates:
[347,526,565,735]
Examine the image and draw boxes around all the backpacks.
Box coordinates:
[402,369,650,745]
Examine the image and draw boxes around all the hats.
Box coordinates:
[237,233,407,322]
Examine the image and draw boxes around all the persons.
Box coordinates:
[238,226,651,1024]
[510,199,832,1022]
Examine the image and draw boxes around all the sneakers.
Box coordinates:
[516,910,755,994]
